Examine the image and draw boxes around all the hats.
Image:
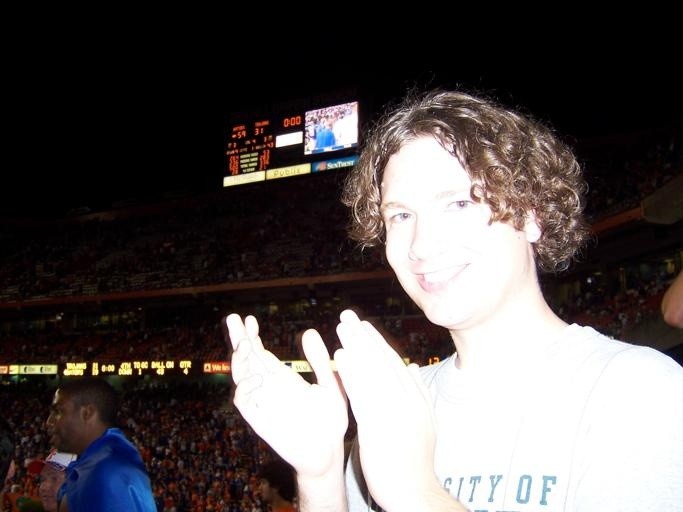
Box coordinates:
[28,450,77,472]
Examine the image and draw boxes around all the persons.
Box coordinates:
[0,88,683,512]
